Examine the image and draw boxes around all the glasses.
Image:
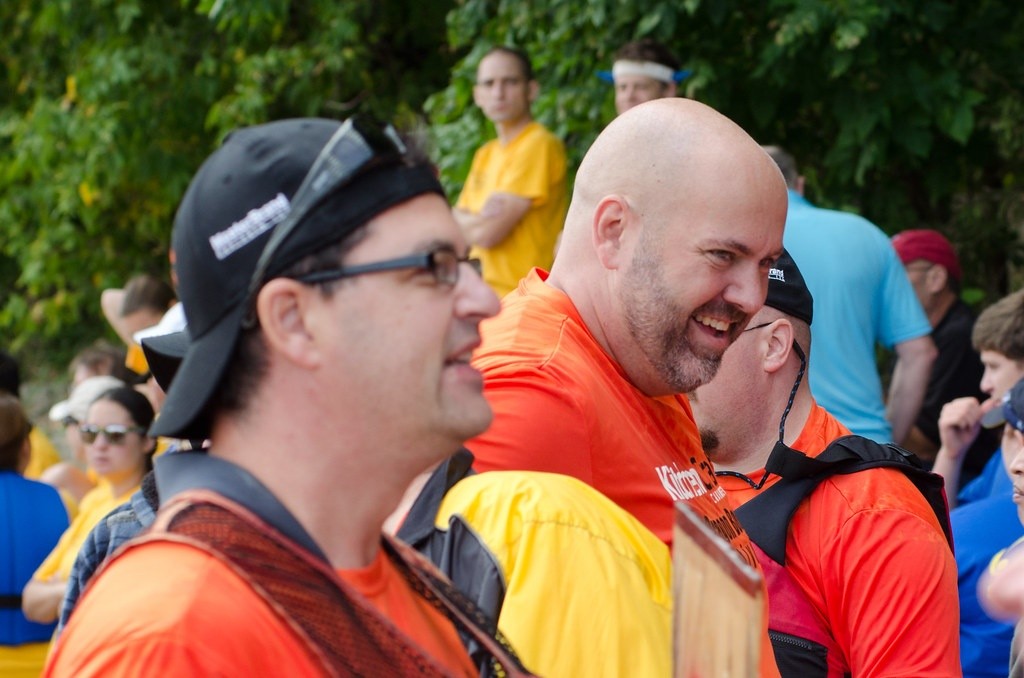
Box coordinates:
[245,246,481,326]
[63,416,78,427]
[249,111,429,293]
[80,424,144,444]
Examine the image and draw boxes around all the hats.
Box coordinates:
[49,375,125,422]
[134,301,188,345]
[141,324,191,393]
[147,115,446,440]
[891,229,956,274]
[980,376,1024,434]
[764,247,814,326]
[101,274,175,344]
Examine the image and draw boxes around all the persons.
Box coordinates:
[38,110,525,678]
[0,39,1024,678]
